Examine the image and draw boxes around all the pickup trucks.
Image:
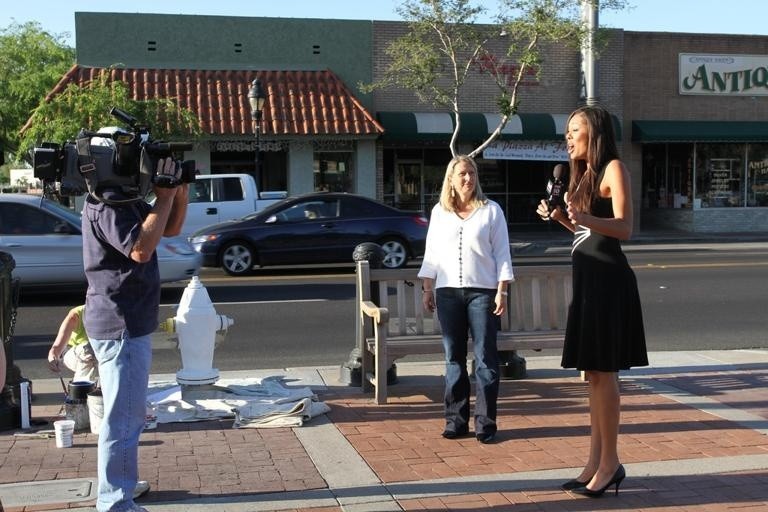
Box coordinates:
[146,172,293,236]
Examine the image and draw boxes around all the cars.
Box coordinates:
[0,191,203,293]
[692,189,754,207]
[185,189,430,279]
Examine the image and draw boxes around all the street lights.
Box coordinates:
[246,76,269,191]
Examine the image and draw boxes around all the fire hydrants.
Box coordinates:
[157,272,237,390]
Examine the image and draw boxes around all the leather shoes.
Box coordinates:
[443,422,469,439]
[476,429,494,443]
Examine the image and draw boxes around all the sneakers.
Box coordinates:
[133,479,150,501]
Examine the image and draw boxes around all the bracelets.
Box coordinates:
[497,290,508,296]
[421,290,434,293]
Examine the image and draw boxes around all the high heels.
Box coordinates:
[571,463,626,497]
[561,477,593,490]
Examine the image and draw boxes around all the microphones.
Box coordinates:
[546,164,567,216]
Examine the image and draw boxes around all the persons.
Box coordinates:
[81,153,188,512]
[416,155,515,444]
[536,107,650,499]
[47,302,99,393]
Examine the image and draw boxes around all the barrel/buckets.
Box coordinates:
[63,401,90,431]
[86,390,104,436]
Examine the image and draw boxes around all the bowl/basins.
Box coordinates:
[68,380,96,400]
